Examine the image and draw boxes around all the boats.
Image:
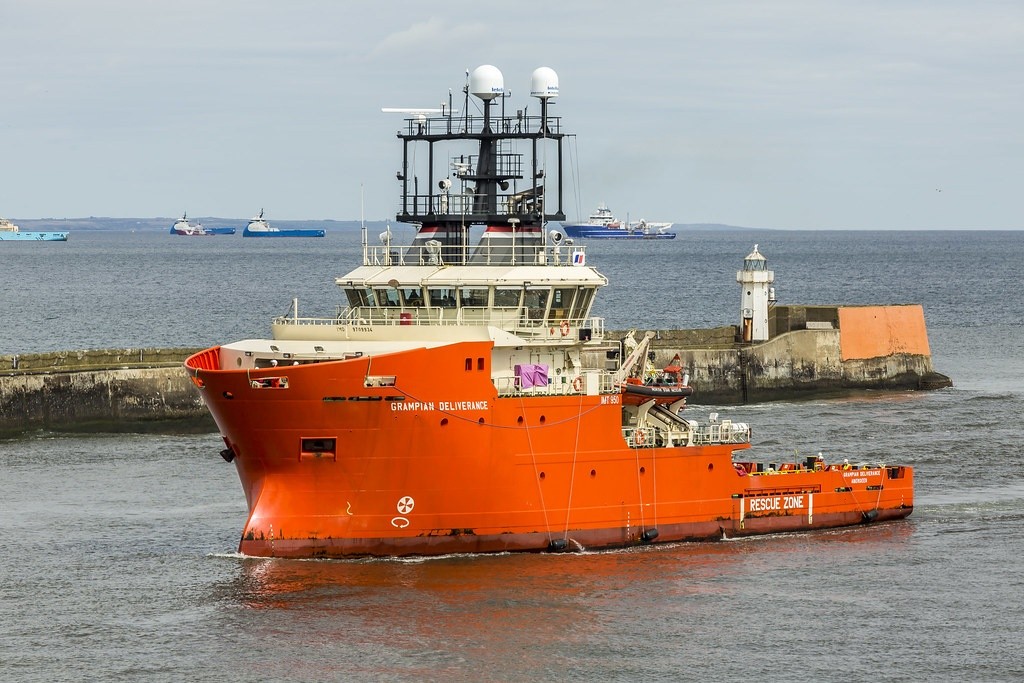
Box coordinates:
[0,216,70,241]
[559,204,676,239]
[243,208,326,237]
[170,211,236,234]
[182,65,913,558]
[175,220,215,235]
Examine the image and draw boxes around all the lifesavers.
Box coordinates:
[560,321,570,336]
[635,430,647,443]
[573,377,582,391]
